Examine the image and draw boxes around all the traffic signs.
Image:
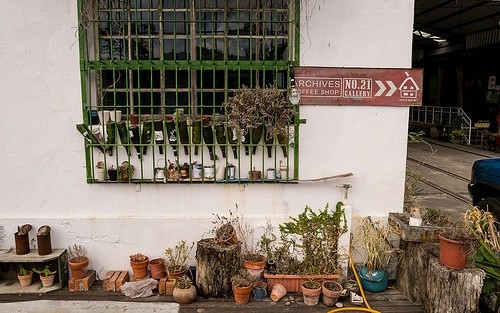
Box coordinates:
[295,67,426,107]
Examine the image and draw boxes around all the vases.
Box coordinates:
[149,258,167,280]
[270,283,287,302]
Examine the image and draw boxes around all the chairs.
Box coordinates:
[474,120,491,147]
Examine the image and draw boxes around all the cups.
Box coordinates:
[110,111,122,123]
[37,225,52,255]
[15,225,32,254]
[97,110,109,125]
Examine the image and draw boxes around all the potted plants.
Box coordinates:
[448,114,469,145]
[95,160,105,182]
[129,202,473,307]
[154,168,164,179]
[193,161,203,179]
[36,225,52,256]
[32,265,57,287]
[228,164,236,180]
[266,168,275,180]
[118,161,135,181]
[60,244,90,279]
[204,165,215,179]
[180,162,189,179]
[279,160,287,179]
[14,224,33,255]
[16,267,34,287]
[108,165,117,181]
[252,167,261,180]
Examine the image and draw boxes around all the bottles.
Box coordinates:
[193,121,201,155]
[141,121,152,155]
[93,128,109,151]
[240,117,287,157]
[203,124,213,159]
[116,121,131,156]
[215,123,226,158]
[77,124,105,154]
[153,120,164,154]
[227,123,237,158]
[165,121,177,156]
[106,122,115,156]
[178,121,189,155]
[129,126,141,159]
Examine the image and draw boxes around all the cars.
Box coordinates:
[465,157,500,228]
